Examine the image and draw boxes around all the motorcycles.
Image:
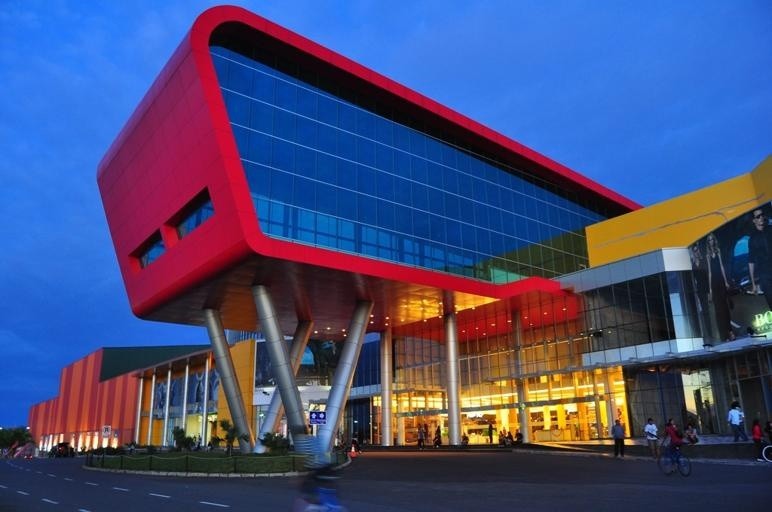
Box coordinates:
[191,435,213,452]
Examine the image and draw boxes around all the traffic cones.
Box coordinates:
[349,443,358,457]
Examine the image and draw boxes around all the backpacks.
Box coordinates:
[674,428,683,438]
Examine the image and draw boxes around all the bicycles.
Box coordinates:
[655,438,694,477]
[763,442,772,463]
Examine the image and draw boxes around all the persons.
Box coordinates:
[461,431,469,444]
[488,421,493,442]
[727,404,746,441]
[417,423,426,450]
[499,430,506,443]
[514,428,523,444]
[506,431,513,447]
[685,424,699,448]
[752,418,764,461]
[353,431,363,454]
[764,421,772,445]
[664,423,683,473]
[643,417,658,455]
[733,400,748,440]
[435,425,442,444]
[130,439,137,455]
[610,419,626,461]
[432,433,441,448]
[668,417,675,427]
[423,418,429,443]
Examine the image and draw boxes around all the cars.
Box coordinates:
[22,453,34,461]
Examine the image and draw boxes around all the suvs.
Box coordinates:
[47,443,77,457]
